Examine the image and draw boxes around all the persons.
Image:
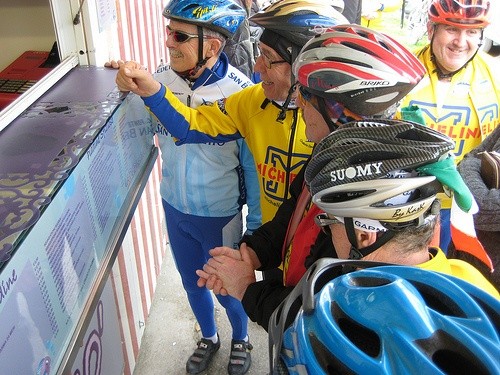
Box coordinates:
[117,0,350,284]
[198,23,426,332]
[106,0,262,375]
[392,0,500,300]
[305,119,500,300]
[267,258,500,375]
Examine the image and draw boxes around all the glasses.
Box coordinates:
[298,86,321,114]
[261,51,287,69]
[166,25,224,43]
[439,0,486,19]
[314,213,342,235]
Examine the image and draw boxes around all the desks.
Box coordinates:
[0,51,53,111]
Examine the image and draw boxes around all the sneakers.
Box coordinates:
[228,335,253,375]
[186,332,221,375]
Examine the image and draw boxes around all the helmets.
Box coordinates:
[304,119,455,223]
[292,23,427,116]
[268,257,500,375]
[162,0,247,41]
[428,0,491,29]
[248,0,351,47]
